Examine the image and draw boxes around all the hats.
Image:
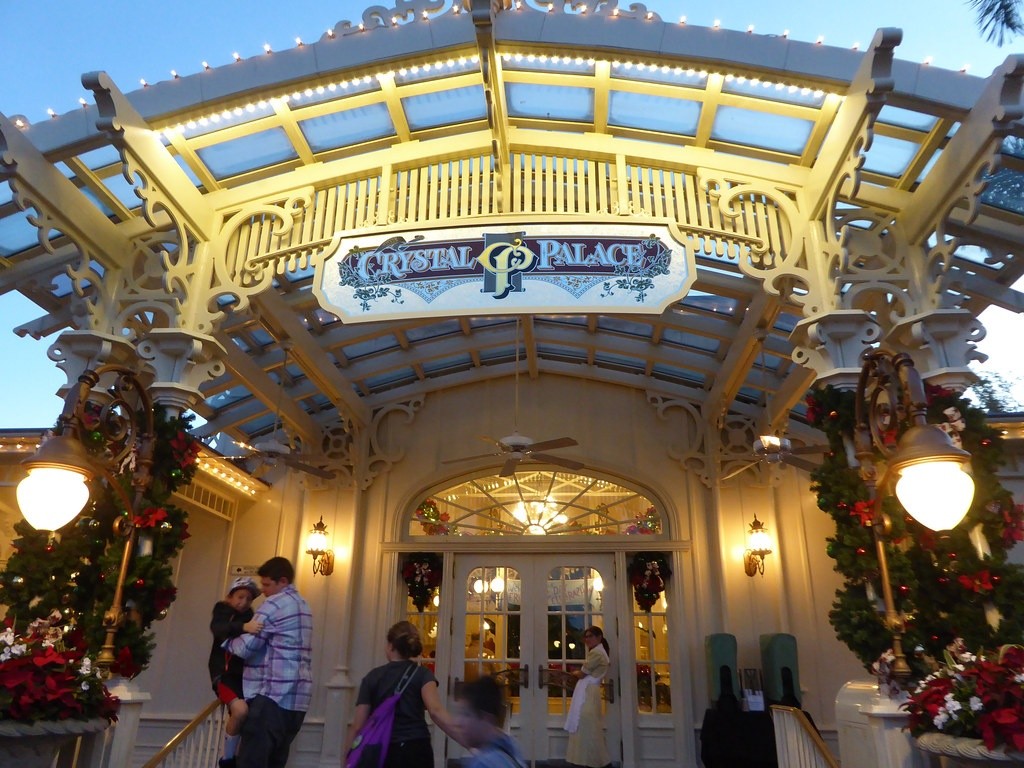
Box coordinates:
[225,577,261,601]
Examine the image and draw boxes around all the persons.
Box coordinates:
[464,633,495,684]
[213,556,314,768]
[207,577,265,768]
[448,677,527,768]
[564,626,612,768]
[341,620,473,768]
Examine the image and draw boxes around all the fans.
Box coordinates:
[197,334,341,487]
[439,314,587,480]
[689,325,837,502]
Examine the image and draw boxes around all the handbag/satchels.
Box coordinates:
[345,692,400,768]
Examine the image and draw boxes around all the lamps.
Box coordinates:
[507,495,571,536]
[14,361,158,683]
[743,516,775,574]
[424,575,505,631]
[851,349,979,689]
[305,514,332,579]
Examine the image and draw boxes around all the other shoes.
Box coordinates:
[218,755,237,768]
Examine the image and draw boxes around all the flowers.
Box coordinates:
[0,617,123,728]
[908,638,1024,751]
